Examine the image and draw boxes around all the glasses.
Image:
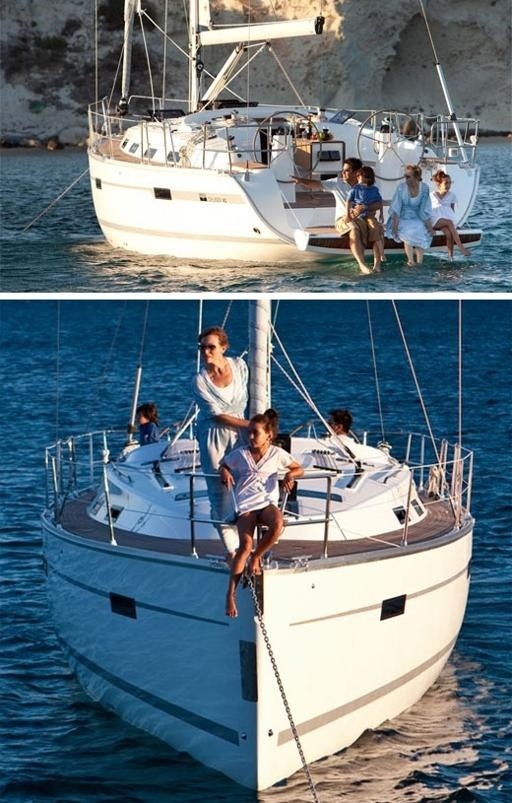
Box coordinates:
[199,344,218,351]
[403,174,411,179]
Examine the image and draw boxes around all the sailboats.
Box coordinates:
[40,300,476,790]
[84,0,484,266]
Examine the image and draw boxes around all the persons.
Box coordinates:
[192,326,255,565]
[327,408,356,443]
[219,406,303,617]
[288,156,382,275]
[136,402,160,446]
[428,168,471,263]
[383,163,435,266]
[346,165,383,247]
